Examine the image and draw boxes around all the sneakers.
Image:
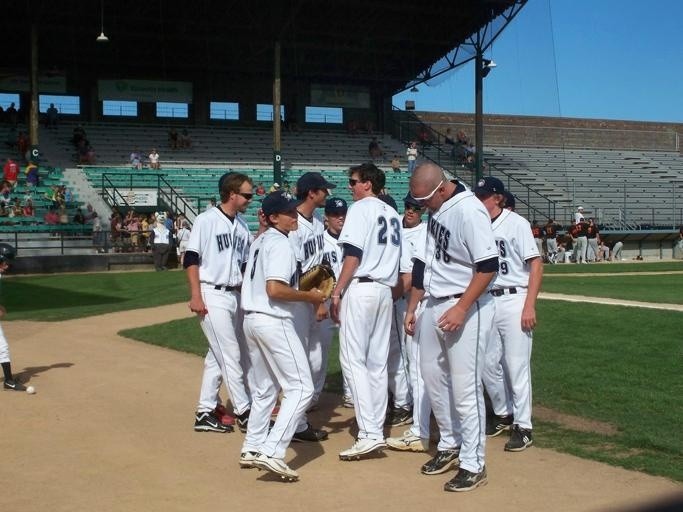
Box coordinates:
[342,395,354,407]
[430,424,440,444]
[384,405,413,427]
[214,404,235,425]
[338,436,389,462]
[233,408,278,434]
[421,446,459,476]
[582,261,587,264]
[442,465,489,493]
[291,422,328,443]
[385,429,430,454]
[237,451,262,468]
[272,406,279,415]
[250,452,300,483]
[485,415,514,438]
[4,379,27,391]
[503,425,535,453]
[576,261,579,264]
[192,411,234,434]
[306,396,320,413]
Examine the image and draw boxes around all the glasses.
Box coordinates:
[409,180,444,203]
[347,177,365,188]
[236,191,254,200]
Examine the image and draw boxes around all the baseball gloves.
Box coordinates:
[298,264,337,302]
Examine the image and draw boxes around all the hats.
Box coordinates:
[294,171,337,190]
[182,220,190,227]
[580,218,583,221]
[260,190,306,215]
[578,207,583,210]
[323,197,347,216]
[0,242,18,265]
[157,215,167,223]
[470,177,506,195]
[503,191,515,211]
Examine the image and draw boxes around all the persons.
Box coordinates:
[384,189,428,428]
[1,243,35,394]
[214,346,281,425]
[206,178,299,210]
[386,225,440,451]
[403,163,501,492]
[0,99,190,171]
[306,198,354,412]
[532,207,624,263]
[474,176,544,451]
[286,172,336,441]
[280,110,477,174]
[0,157,192,272]
[182,173,276,432]
[239,190,327,481]
[331,163,402,460]
